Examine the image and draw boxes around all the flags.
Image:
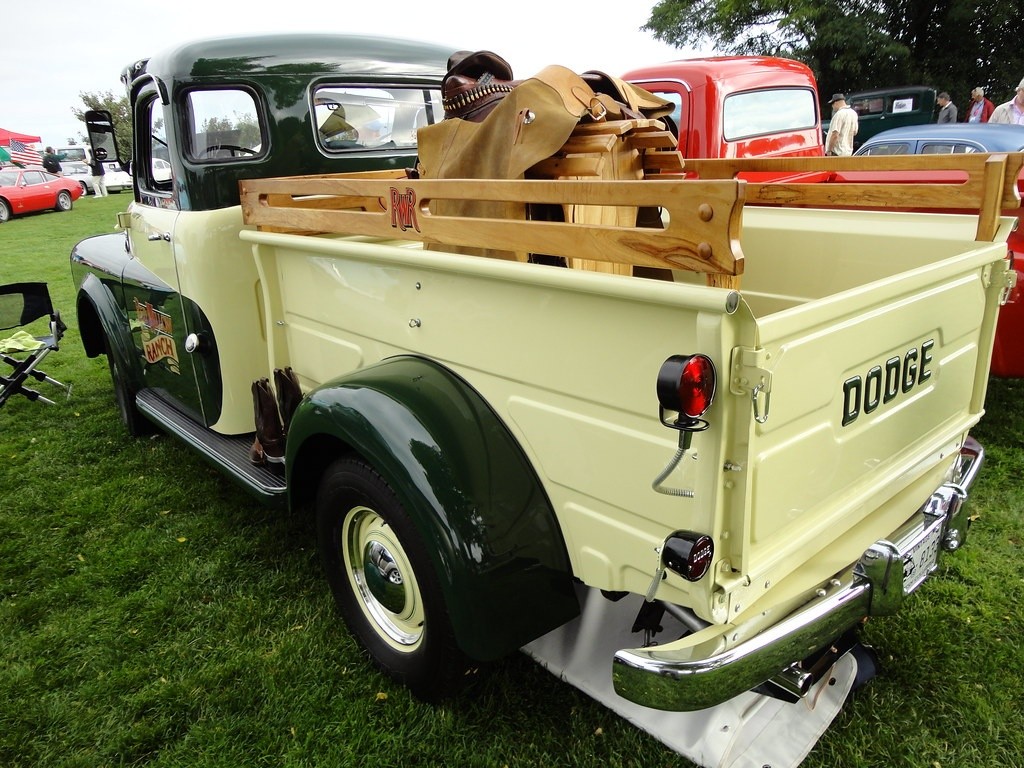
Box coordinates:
[8,138,45,166]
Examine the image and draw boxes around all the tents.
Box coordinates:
[0,128,42,147]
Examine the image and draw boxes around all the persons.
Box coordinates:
[81,149,108,200]
[936,92,958,124]
[964,87,995,125]
[822,93,860,159]
[989,76,1024,128]
[42,146,68,179]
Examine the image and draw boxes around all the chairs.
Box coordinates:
[0,281,74,412]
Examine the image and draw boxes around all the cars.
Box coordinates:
[37,146,172,196]
[854,123,1023,156]
[0,169,82,222]
[822,87,937,151]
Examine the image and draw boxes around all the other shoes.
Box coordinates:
[93,195,102,198]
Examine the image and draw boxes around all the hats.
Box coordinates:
[827,94,845,103]
[441,50,514,98]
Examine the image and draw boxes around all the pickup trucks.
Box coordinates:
[618,55,1023,379]
[69,32,1024,768]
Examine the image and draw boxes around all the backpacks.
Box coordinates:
[44,157,59,173]
